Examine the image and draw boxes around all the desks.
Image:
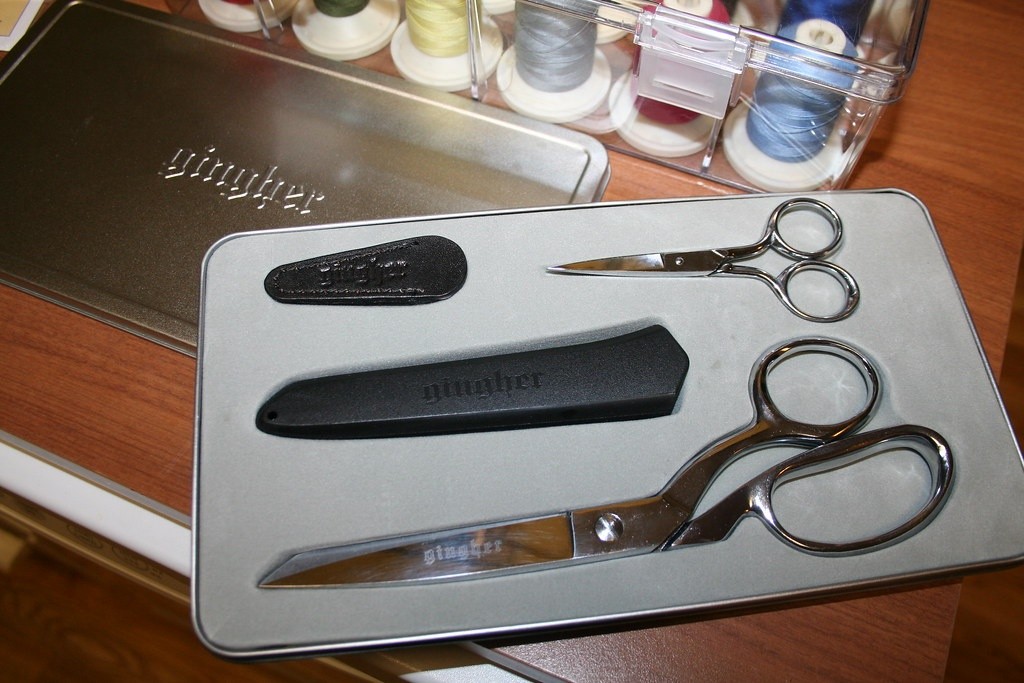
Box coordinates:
[0,0,1024,683]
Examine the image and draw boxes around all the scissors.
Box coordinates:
[256,333,957,595]
[547,197,859,322]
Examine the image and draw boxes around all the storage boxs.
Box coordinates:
[164,0,929,193]
